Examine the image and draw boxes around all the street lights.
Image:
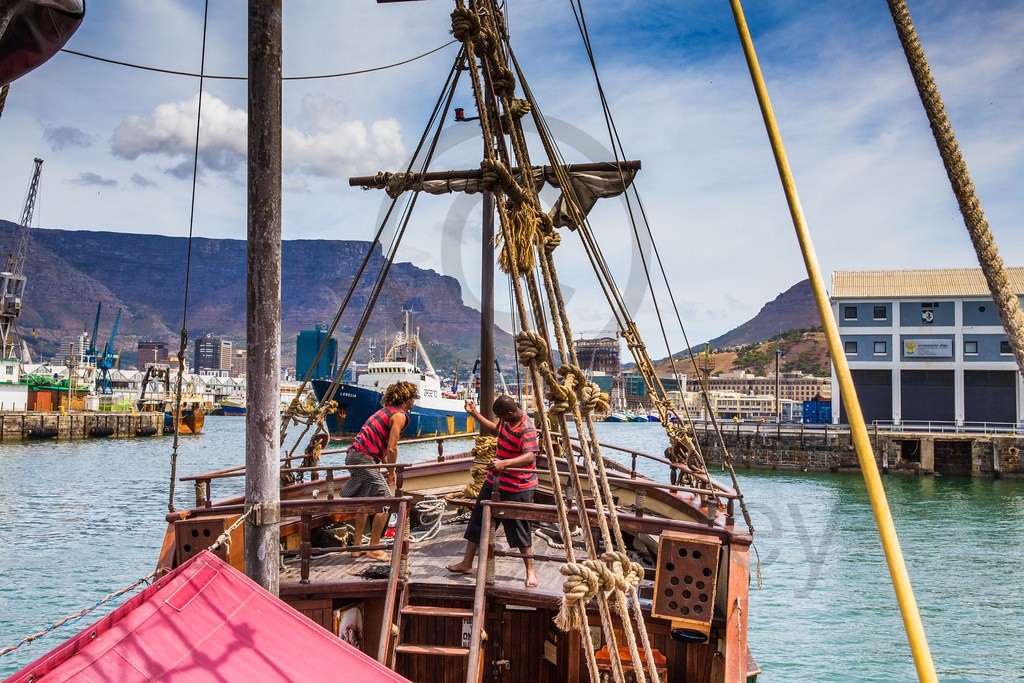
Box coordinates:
[775,346,786,426]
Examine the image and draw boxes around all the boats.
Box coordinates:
[672,390,804,427]
[218,399,289,416]
[647,407,677,422]
[139,0,763,683]
[635,402,649,422]
[309,302,480,440]
[604,408,627,421]
[625,408,635,422]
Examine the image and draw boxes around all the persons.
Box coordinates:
[345,381,420,562]
[446,395,539,587]
